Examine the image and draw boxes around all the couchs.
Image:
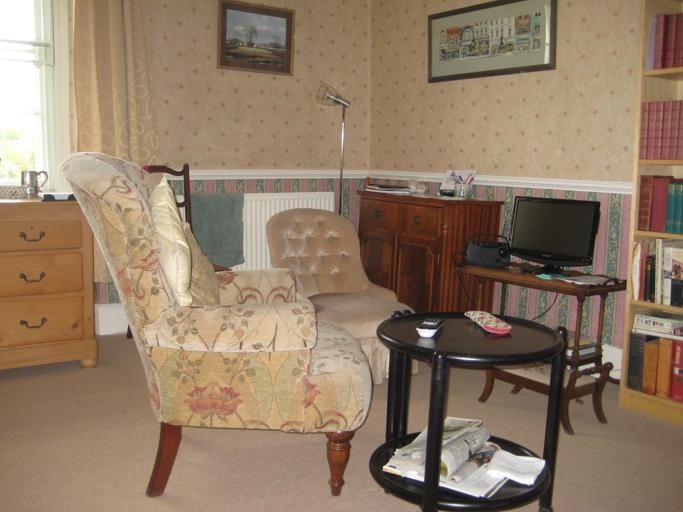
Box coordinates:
[266,209,417,385]
[59,151,373,498]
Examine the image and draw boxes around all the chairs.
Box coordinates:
[127,163,191,338]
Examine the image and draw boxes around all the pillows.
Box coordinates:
[145,175,220,312]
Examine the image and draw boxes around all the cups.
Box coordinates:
[20,171,47,197]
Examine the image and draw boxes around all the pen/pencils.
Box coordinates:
[449,168,474,184]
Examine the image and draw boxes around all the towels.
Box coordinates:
[190,191,244,268]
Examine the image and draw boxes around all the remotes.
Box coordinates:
[415,317,445,338]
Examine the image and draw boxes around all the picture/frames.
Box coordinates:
[428,0,556,84]
[217,1,294,76]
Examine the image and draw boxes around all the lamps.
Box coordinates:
[313,81,351,216]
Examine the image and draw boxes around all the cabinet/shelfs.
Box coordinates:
[0,197,98,371]
[453,263,625,436]
[616,0,682,426]
[355,190,504,313]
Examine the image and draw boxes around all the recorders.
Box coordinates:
[463,233,510,269]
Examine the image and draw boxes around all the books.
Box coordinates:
[640,101,683,160]
[627,313,683,408]
[646,11,683,71]
[639,176,682,234]
[631,238,683,307]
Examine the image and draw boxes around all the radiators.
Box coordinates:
[173,192,335,272]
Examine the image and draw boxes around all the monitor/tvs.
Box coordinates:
[508,195,600,275]
